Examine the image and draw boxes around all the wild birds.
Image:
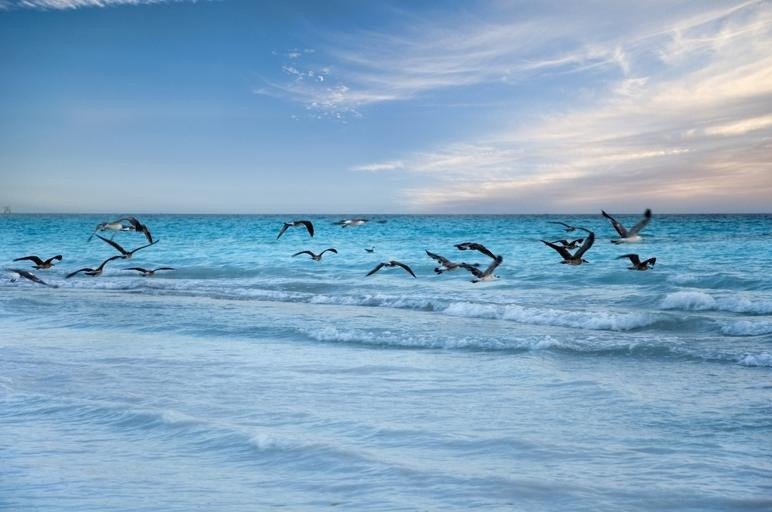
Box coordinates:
[13,255,62,270]
[290,247,338,261]
[601,208,652,246]
[364,260,417,279]
[335,218,369,228]
[8,267,47,286]
[546,219,590,233]
[425,240,502,284]
[87,216,159,258]
[540,231,594,266]
[614,253,657,272]
[65,255,128,279]
[122,267,177,277]
[277,219,314,241]
[364,246,376,252]
